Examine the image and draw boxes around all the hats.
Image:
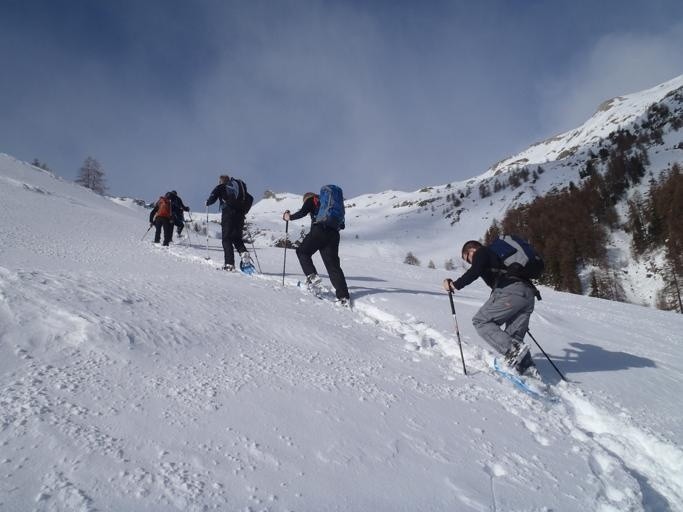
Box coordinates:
[303,192,315,203]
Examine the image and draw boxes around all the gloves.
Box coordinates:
[282,212,291,222]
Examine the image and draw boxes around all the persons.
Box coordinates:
[283,192,352,309]
[442,235,544,376]
[170,189,190,237]
[205,173,255,273]
[150,192,183,248]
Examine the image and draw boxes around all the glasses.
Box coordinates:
[466,253,472,264]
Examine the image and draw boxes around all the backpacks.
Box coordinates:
[226,178,254,213]
[157,196,172,218]
[316,184,346,230]
[487,233,545,278]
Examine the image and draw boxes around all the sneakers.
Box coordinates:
[222,264,236,272]
[519,365,544,382]
[504,339,531,370]
[241,252,251,267]
[337,296,351,306]
[305,273,324,288]
